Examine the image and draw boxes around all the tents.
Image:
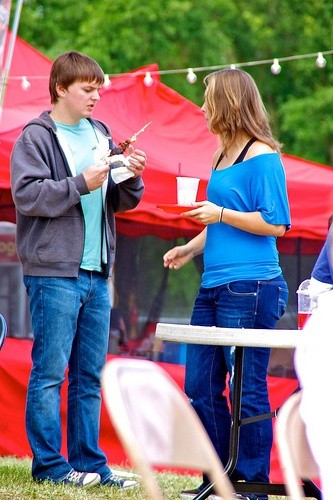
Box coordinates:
[0,24,79,225]
[1,61,333,491]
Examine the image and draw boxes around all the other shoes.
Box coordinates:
[180,484,215,499]
[205,492,253,500]
[60,470,101,488]
[100,474,140,491]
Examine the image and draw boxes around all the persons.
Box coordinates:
[161,66,290,500]
[8,51,147,490]
[309,206,333,325]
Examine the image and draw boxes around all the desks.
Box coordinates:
[156,321,321,500]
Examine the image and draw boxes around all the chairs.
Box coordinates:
[99,358,240,500]
[274,389,321,500]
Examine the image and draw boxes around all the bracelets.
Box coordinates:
[219,206,225,223]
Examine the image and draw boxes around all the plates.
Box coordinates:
[156,204,204,214]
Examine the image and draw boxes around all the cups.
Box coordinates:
[176,177,201,204]
[296,279,317,330]
